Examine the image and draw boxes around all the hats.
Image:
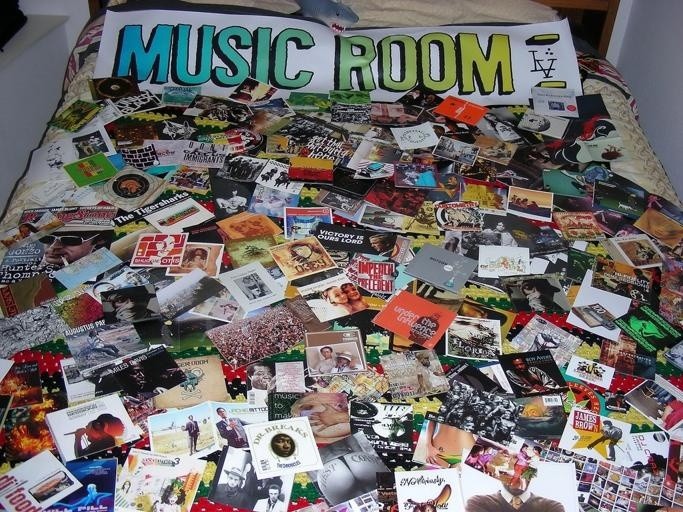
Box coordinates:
[336,350,352,362]
[521,279,560,292]
[226,467,244,480]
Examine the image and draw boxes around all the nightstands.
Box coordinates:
[0,12,72,225]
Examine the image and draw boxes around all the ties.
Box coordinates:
[513,496,522,509]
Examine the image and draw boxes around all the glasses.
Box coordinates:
[40,233,101,245]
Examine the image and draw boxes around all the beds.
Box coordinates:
[0,0,683,275]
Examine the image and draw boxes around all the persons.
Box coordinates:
[0,75,680,511]
